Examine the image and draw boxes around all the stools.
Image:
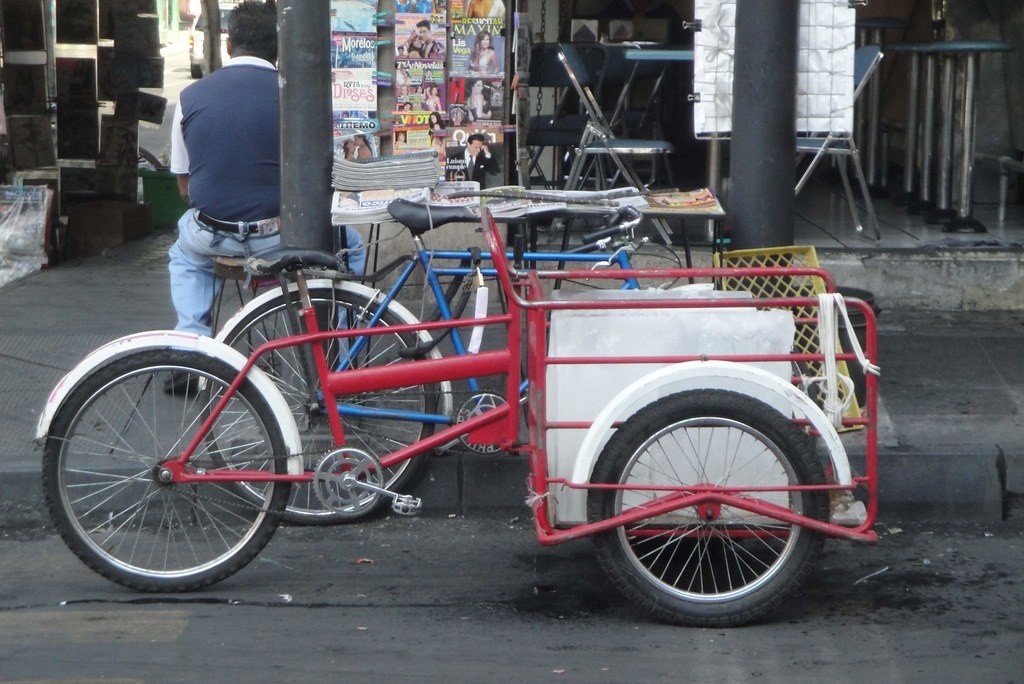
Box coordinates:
[211,257,279,384]
[854,17,1016,236]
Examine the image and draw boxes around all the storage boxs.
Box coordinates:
[68,201,153,254]
[546,288,796,525]
[137,169,191,230]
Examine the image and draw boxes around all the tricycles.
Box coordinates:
[34,201,879,630]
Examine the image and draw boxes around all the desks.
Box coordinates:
[350,188,727,424]
[625,48,719,241]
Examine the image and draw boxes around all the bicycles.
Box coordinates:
[197,201,683,526]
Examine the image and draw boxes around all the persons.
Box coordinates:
[398,66,442,112]
[396,15,446,59]
[465,0,506,20]
[395,131,406,143]
[343,134,372,161]
[468,31,499,76]
[445,134,501,191]
[467,78,491,123]
[164,0,380,404]
[428,112,445,153]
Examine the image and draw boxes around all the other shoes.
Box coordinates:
[163,369,199,392]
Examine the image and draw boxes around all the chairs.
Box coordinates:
[522,42,677,246]
[794,43,883,241]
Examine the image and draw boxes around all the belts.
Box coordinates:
[198,213,258,233]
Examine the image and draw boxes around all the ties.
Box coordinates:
[467,155,474,180]
